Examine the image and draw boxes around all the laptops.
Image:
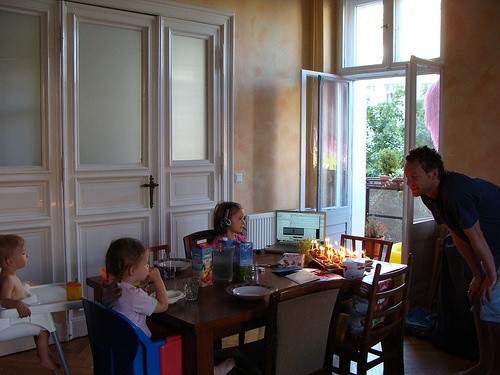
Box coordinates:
[264,210,326,254]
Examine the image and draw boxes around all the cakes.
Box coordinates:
[309,246,372,269]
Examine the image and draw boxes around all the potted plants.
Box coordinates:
[378,145,404,190]
[363,214,385,256]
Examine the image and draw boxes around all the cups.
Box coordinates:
[342,258,365,279]
[66,282,82,301]
[163,253,176,277]
[182,276,199,301]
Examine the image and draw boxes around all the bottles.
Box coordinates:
[212,237,235,281]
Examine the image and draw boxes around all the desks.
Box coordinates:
[87,239,408,375]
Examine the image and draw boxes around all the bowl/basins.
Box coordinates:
[153,258,192,273]
[225,282,278,300]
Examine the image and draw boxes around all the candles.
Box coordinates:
[311,236,355,264]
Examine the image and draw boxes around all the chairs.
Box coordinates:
[0,282,84,375]
[340,234,393,262]
[222,278,347,375]
[432,233,480,360]
[181,229,216,259]
[326,251,415,375]
[81,296,183,375]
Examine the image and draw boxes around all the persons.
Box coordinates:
[0,233,60,375]
[405,145,500,375]
[102,238,236,375]
[209,201,247,246]
